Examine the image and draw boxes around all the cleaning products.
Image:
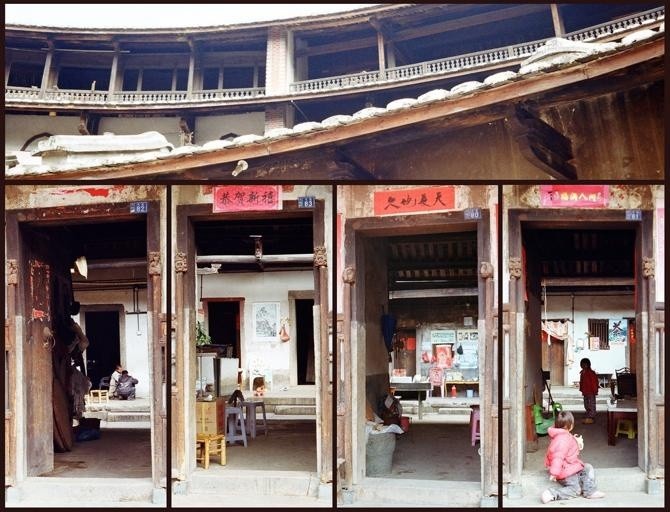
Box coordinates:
[452,385,457,397]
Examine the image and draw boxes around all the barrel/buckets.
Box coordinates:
[401,416,409,433]
[590,337,600,350]
[466,389,473,397]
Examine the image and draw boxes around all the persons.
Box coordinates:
[579,358,599,424]
[116,369,139,400]
[540,410,607,504]
[109,365,123,400]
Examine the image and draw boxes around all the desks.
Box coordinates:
[391,376,479,396]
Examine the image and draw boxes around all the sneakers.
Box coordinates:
[584,490,606,499]
[582,416,595,425]
[540,487,556,504]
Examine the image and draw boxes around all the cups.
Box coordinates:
[611,397,618,404]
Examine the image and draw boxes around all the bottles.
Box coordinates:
[205,384,213,392]
[573,433,583,450]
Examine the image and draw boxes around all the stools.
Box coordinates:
[90,378,110,403]
[470,408,480,447]
[608,369,637,446]
[197,396,266,467]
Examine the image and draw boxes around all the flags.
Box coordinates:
[533,401,563,436]
